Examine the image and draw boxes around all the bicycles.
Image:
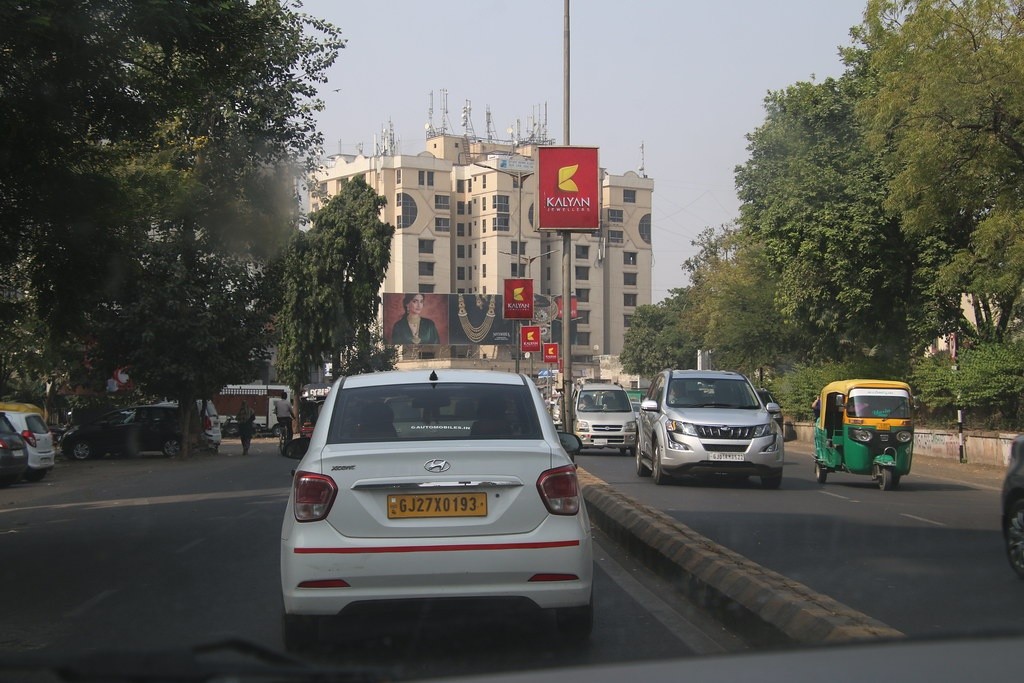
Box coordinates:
[279,418,296,454]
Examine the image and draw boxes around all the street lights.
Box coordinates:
[473,162,534,373]
[533,291,574,398]
[546,317,584,373]
[499,249,560,376]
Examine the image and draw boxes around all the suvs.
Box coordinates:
[634,368,785,488]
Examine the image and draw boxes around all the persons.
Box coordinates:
[235,400,256,455]
[392,293,440,344]
[862,396,889,417]
[579,394,594,410]
[812,396,820,420]
[275,392,296,442]
[668,384,684,404]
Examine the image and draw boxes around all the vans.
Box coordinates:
[571,382,637,456]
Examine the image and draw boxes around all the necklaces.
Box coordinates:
[407,316,421,344]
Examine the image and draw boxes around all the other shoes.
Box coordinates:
[243,449,248,455]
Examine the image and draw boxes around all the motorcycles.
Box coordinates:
[811,379,916,491]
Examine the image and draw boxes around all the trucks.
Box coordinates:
[220,397,284,438]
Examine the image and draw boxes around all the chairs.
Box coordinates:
[602,396,617,407]
[470,396,512,438]
[358,402,400,437]
[714,384,735,402]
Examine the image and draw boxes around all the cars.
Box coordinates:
[1001,436,1024,579]
[0,411,55,484]
[755,388,783,432]
[124,400,222,446]
[279,368,595,651]
[59,404,180,460]
[550,397,562,430]
[0,412,29,487]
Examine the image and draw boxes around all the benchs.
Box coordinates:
[824,412,863,467]
